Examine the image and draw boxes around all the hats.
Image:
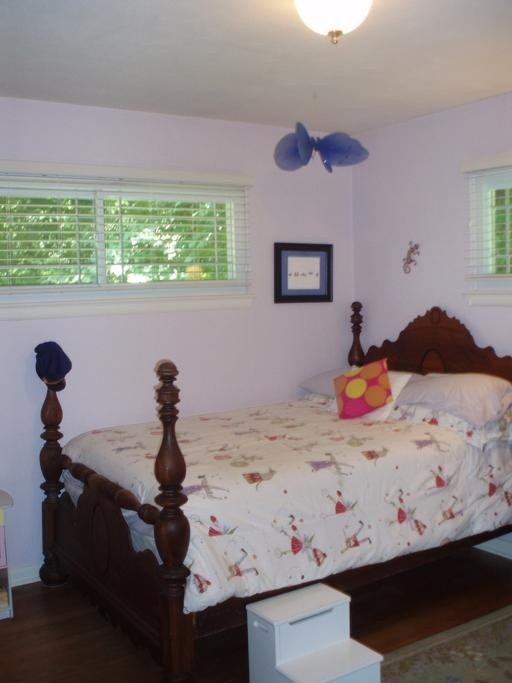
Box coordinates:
[32,340,72,393]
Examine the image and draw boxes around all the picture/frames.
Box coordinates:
[272,241,336,305]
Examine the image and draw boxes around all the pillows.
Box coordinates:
[298,357,512,452]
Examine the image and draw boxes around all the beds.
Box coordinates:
[34,299,512,680]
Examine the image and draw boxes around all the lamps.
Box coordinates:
[294,0,373,43]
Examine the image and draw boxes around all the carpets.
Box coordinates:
[378,603,511,682]
